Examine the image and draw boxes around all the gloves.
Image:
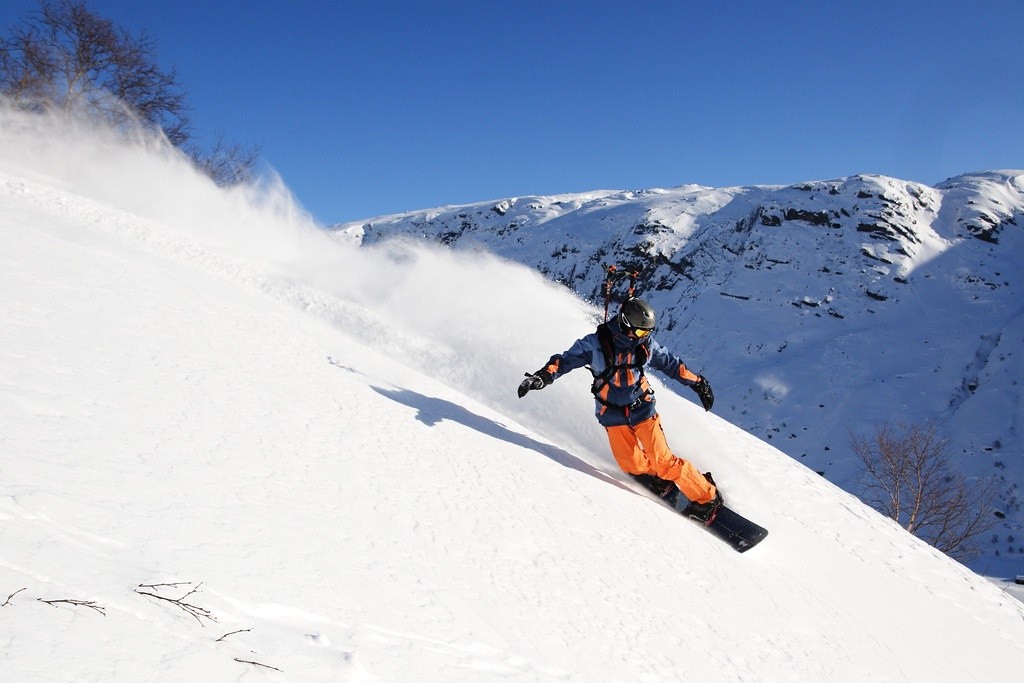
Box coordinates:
[689,375,714,412]
[518,369,553,398]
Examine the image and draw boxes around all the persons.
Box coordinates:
[519,300,724,525]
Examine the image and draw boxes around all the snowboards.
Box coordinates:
[628,464,769,552]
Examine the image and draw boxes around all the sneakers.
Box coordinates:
[687,471,724,527]
[645,472,675,497]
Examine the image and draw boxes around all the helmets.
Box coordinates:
[619,297,656,336]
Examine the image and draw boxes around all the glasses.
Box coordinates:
[631,327,651,338]
